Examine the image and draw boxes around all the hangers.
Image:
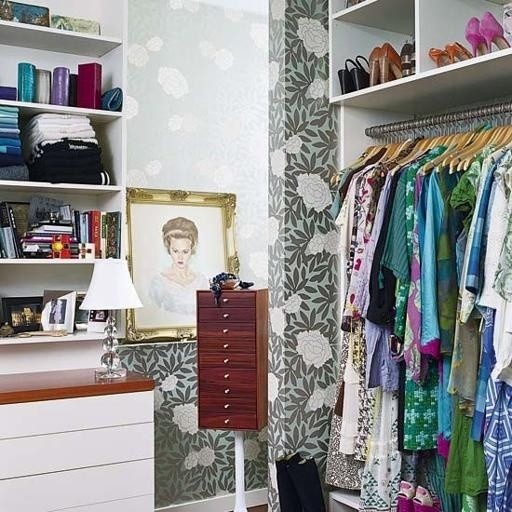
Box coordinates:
[325,97,510,187]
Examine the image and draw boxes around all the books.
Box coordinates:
[1,199,122,259]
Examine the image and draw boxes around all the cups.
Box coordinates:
[79,243,96,259]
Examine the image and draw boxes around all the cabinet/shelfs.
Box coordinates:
[0,1,129,348]
[0,390,156,512]
[195,286,270,511]
[326,0,512,107]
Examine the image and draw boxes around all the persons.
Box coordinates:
[146,215,211,326]
[51,299,56,310]
[52,298,64,324]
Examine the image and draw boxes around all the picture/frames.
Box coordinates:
[0,288,77,335]
[123,186,241,348]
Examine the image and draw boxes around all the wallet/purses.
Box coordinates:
[101,88,122,111]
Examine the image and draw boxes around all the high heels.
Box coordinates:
[397,480,440,512]
[400,41,416,77]
[379,42,402,84]
[465,12,511,58]
[429,42,452,68]
[338,55,357,95]
[446,42,473,62]
[351,56,369,91]
[369,42,381,86]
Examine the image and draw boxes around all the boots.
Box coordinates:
[275,452,327,512]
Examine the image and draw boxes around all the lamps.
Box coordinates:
[78,258,144,383]
